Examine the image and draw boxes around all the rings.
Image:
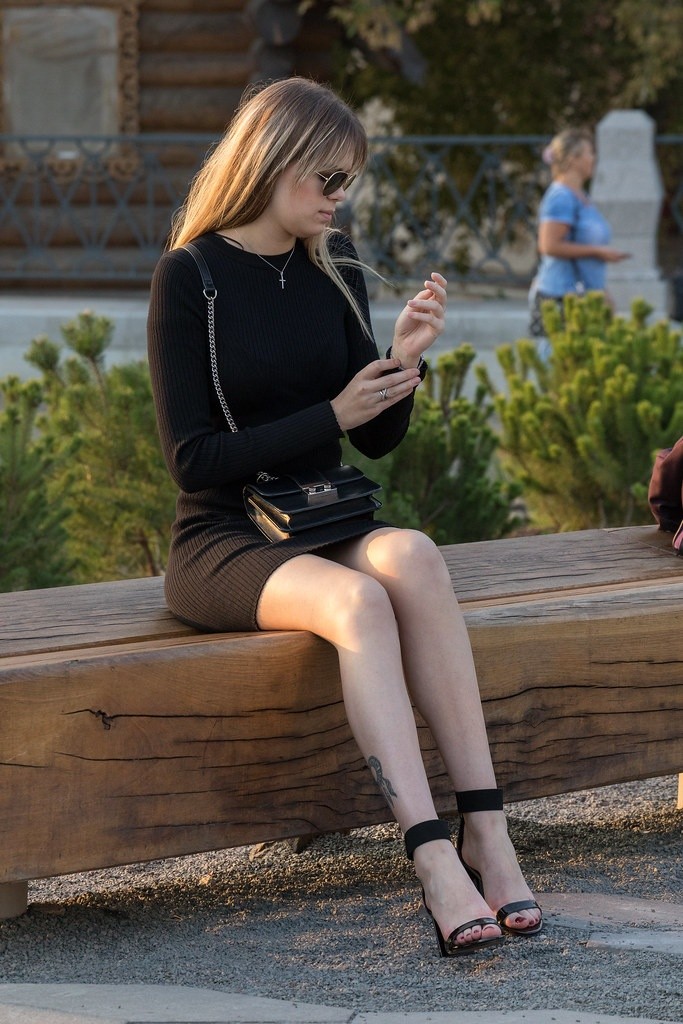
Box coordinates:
[379,389,388,400]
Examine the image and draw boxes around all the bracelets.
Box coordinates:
[390,348,425,371]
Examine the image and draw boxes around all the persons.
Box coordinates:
[524,130,635,337]
[653,143,683,323]
[145,77,546,956]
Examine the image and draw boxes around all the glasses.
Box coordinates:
[295,156,356,197]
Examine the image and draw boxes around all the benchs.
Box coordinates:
[0,524,683,918]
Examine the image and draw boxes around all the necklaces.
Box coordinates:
[234,228,295,289]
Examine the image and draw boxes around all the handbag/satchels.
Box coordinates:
[241,463,384,544]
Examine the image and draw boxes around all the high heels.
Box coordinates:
[453,788,544,934]
[404,817,506,955]
